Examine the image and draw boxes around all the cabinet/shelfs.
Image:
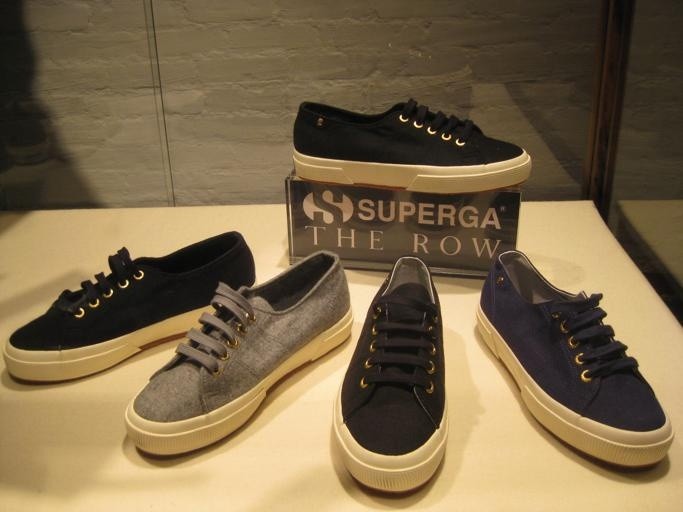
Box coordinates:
[0,199,683,512]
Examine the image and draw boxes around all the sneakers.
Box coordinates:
[292,99,533,194]
[4,229,256,385]
[332,256,448,498]
[477,251,674,473]
[125,252,353,458]
